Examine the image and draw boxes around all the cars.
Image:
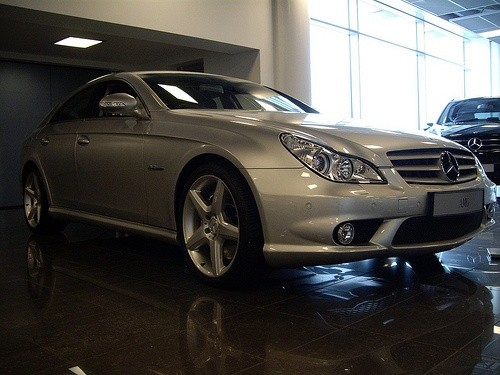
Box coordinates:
[428,95,500,187]
[16,70,497,288]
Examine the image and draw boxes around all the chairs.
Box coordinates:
[457,108,474,120]
